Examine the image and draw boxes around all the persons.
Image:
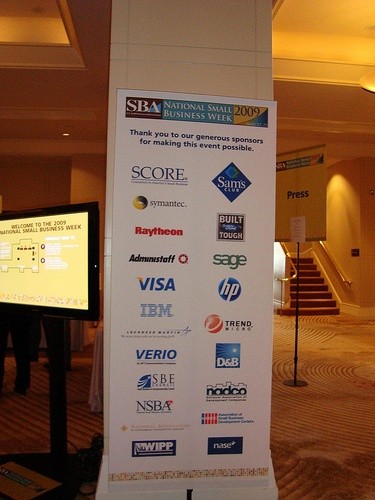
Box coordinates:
[27,314,50,369]
[0,309,31,398]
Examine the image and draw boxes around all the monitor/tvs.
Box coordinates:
[0,201,100,321]
